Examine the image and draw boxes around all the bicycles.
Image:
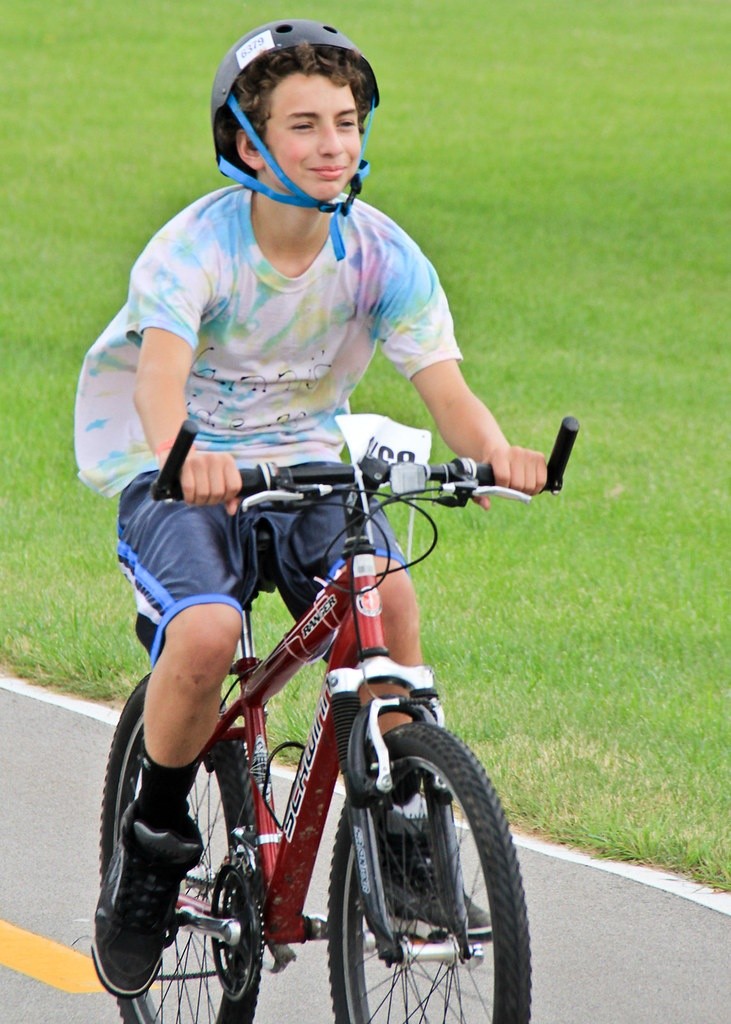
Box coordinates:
[95,416,583,1023]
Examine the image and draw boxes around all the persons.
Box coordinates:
[70,29,547,997]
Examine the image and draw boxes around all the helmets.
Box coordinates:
[210,19,382,176]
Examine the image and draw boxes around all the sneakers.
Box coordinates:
[375,809,493,945]
[90,799,203,999]
